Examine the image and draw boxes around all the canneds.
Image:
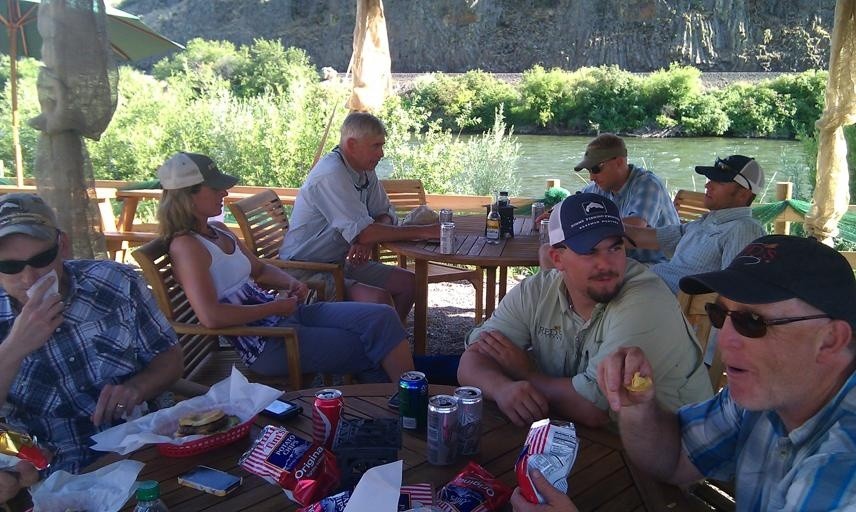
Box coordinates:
[453,386,483,457]
[440,222,455,255]
[426,395,460,467]
[397,371,428,435]
[531,201,545,230]
[438,208,453,223]
[538,218,550,244]
[312,389,344,448]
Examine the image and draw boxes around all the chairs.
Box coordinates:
[673,282,741,395]
[129,225,326,407]
[670,190,714,226]
[226,188,349,305]
[369,179,484,326]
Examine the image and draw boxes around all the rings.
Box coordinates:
[116,402,125,408]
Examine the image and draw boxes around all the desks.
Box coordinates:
[376,211,665,362]
[24,382,741,511]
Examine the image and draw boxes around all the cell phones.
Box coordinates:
[388,387,400,412]
[262,396,303,421]
[180,463,243,495]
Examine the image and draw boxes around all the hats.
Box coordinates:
[158,151,239,192]
[0,194,59,243]
[573,133,629,171]
[548,190,638,255]
[679,234,855,325]
[401,205,440,242]
[695,154,765,195]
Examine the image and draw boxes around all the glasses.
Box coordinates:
[587,157,615,175]
[0,231,60,274]
[714,156,752,190]
[705,302,832,338]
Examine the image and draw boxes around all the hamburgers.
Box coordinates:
[173,410,240,439]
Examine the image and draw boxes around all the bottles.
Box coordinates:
[538,219,551,248]
[485,191,503,245]
[497,191,510,207]
[130,479,170,512]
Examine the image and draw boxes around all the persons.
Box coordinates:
[638,154,770,293]
[599,232,855,512]
[456,188,716,430]
[534,135,683,264]
[280,111,440,323]
[156,152,416,383]
[0,191,186,478]
